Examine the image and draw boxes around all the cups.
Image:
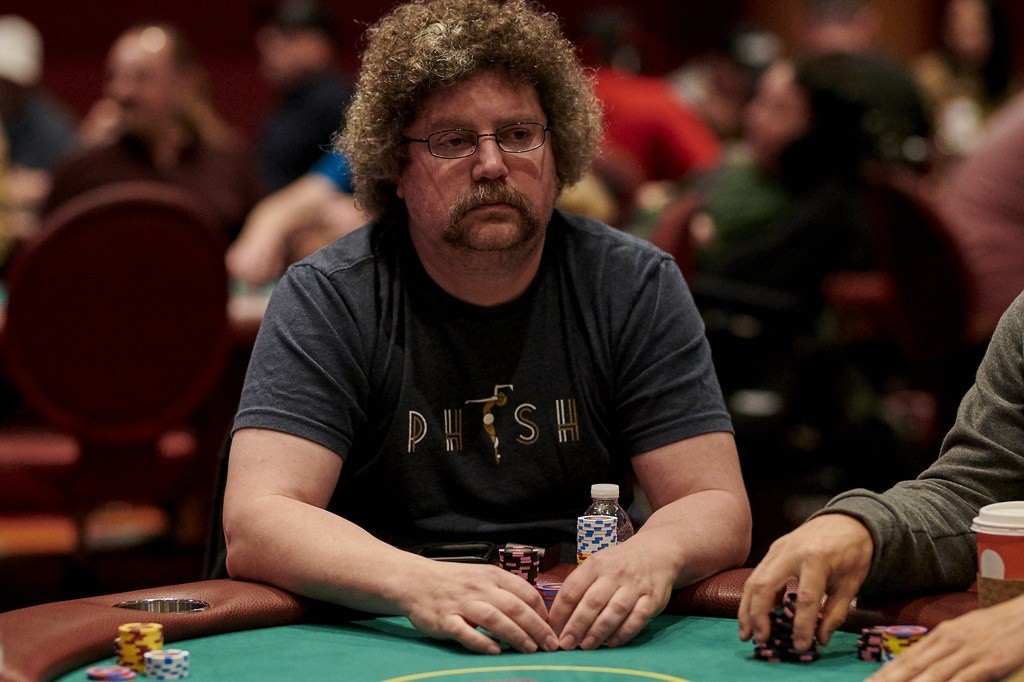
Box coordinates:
[971,501,1024,609]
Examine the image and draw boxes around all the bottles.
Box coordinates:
[583,483,634,544]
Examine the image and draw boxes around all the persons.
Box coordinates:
[0,0,370,284]
[221,0,752,653]
[738,288,1024,682]
[564,0,1024,416]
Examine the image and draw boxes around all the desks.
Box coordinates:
[0,544,984,682]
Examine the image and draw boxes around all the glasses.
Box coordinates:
[401,123,551,159]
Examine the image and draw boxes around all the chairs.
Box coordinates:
[704,187,964,498]
[1,177,233,556]
